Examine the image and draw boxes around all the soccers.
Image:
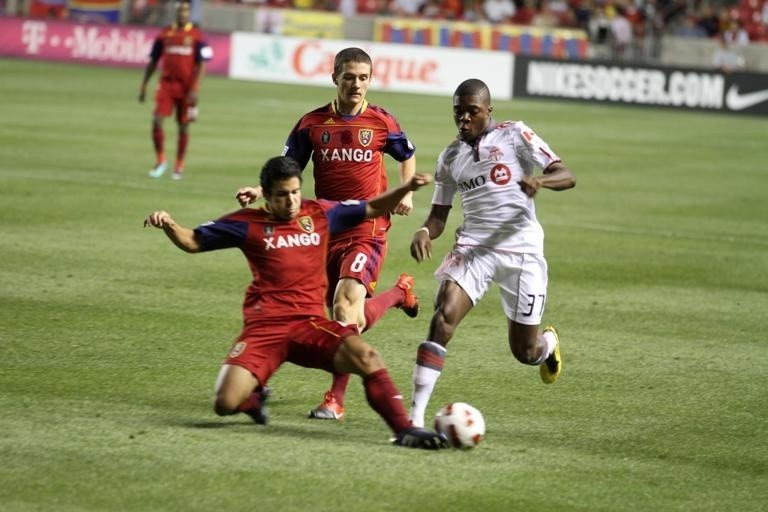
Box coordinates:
[434,402,486,449]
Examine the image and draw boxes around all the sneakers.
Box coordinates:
[308,391,344,420]
[244,386,269,425]
[395,273,419,318]
[539,326,562,385]
[148,162,181,180]
[390,427,448,450]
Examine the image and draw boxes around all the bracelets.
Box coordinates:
[418,226,430,234]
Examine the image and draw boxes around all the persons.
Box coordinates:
[139,1,215,179]
[407,78,578,429]
[235,47,420,422]
[143,155,450,449]
